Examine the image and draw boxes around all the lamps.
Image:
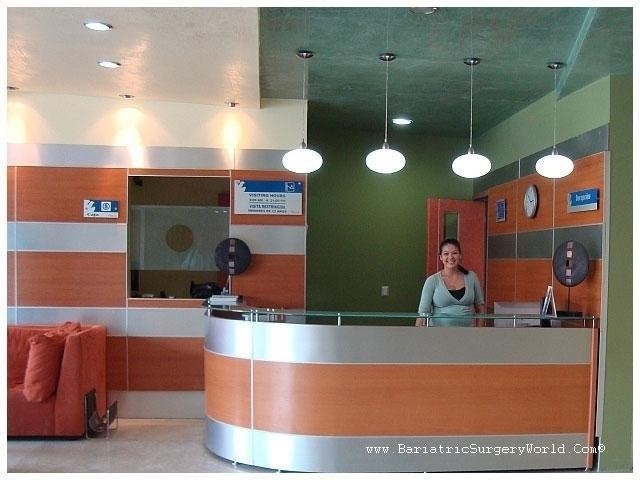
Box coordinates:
[450,57,492,180]
[391,118,413,125]
[365,53,406,174]
[535,62,575,180]
[281,51,324,174]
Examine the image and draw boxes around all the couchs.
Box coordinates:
[7,324,108,438]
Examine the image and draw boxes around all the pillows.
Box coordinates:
[22,322,82,404]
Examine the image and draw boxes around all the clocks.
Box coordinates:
[522,184,539,219]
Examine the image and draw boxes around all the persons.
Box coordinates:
[414,239,486,326]
